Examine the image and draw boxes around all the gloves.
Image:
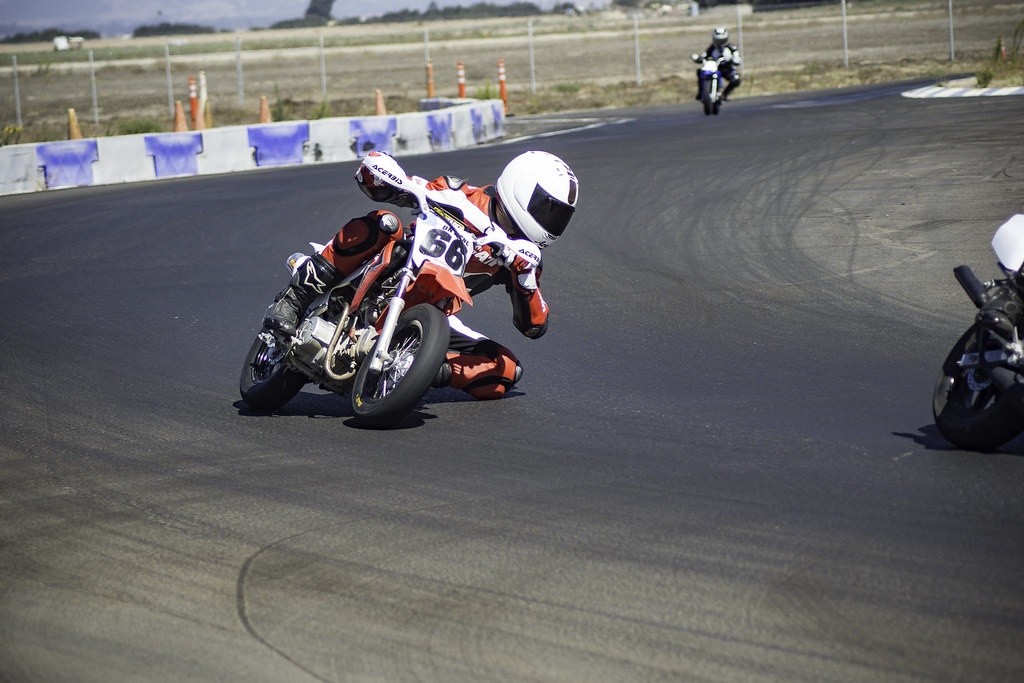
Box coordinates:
[503,239,543,272]
[354,150,396,187]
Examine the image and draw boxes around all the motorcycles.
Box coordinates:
[239,155,512,431]
[696,56,735,117]
[931,265,1024,452]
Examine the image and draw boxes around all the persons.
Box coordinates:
[265,150,579,401]
[694,28,743,102]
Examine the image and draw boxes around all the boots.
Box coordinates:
[430,360,452,388]
[262,251,339,336]
[975,260,1024,337]
[721,82,736,101]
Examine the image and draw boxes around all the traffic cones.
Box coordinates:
[375,89,389,116]
[258,96,275,124]
[173,100,189,133]
[66,106,82,141]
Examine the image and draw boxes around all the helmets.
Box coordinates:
[497,151,579,250]
[712,27,730,47]
